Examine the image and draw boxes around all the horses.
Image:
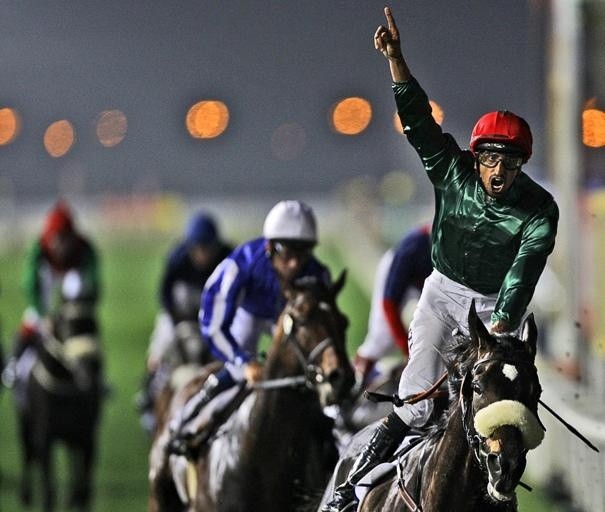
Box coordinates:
[148,280,212,410]
[13,279,102,512]
[343,355,407,432]
[145,264,358,512]
[314,297,544,512]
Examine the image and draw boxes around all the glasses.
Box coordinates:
[272,241,312,259]
[476,150,524,170]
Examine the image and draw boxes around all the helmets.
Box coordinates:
[263,199,318,244]
[185,209,221,245]
[470,110,532,163]
[39,199,76,252]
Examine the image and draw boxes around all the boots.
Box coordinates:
[321,427,402,511]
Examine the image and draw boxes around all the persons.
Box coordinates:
[351,220,433,405]
[0,198,117,403]
[131,207,234,434]
[162,199,331,445]
[326,6,560,510]
